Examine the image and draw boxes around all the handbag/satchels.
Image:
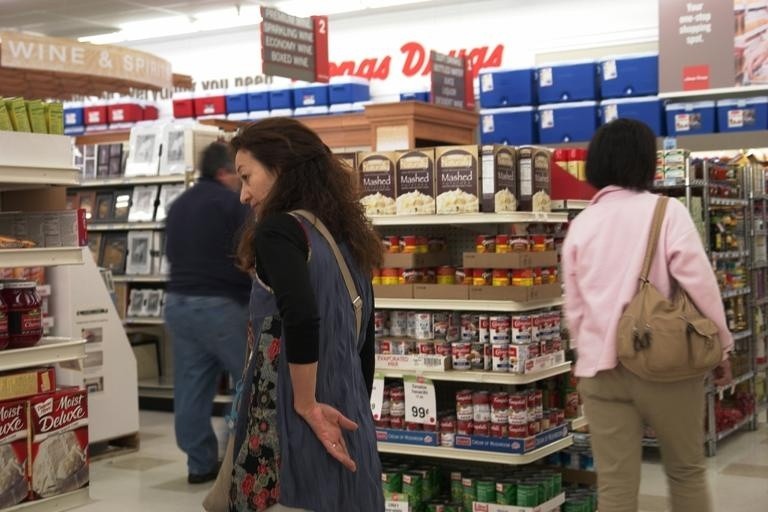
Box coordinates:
[203,433,236,511]
[616,195,723,383]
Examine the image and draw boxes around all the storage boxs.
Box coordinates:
[398,76,431,101]
[712,96,768,133]
[170,76,370,120]
[478,52,663,146]
[662,98,716,136]
[62,94,159,129]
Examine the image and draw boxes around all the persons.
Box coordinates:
[203,115,384,512]
[562,118,734,512]
[163,141,256,483]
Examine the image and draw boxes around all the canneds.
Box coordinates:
[380,456,598,512]
[371,235,558,286]
[375,309,570,372]
[0,278,43,350]
[376,379,582,438]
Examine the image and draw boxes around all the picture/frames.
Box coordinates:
[125,121,193,317]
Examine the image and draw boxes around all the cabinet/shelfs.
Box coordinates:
[1,131,98,512]
[71,116,238,404]
[641,159,755,457]
[756,160,766,407]
[368,211,598,512]
[552,199,599,454]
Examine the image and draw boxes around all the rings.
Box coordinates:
[331,443,337,448]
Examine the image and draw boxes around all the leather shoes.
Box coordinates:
[188,461,222,483]
